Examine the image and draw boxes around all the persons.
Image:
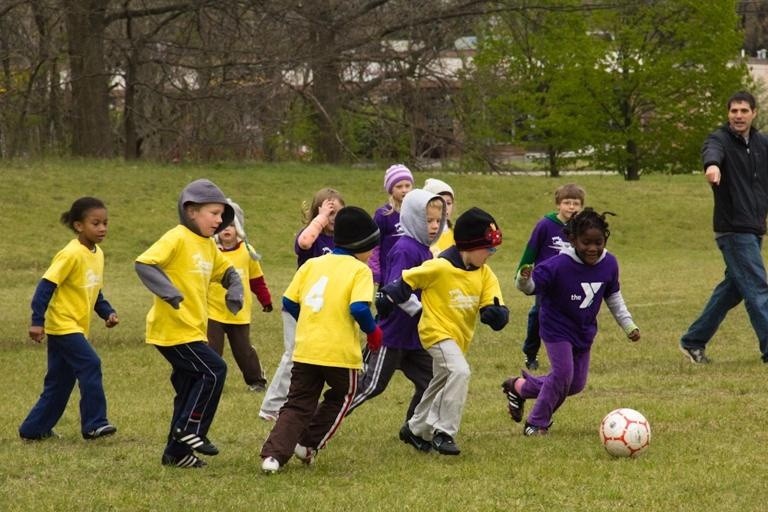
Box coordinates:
[379,206,510,458]
[343,190,447,453]
[679,92,768,362]
[258,204,386,473]
[501,206,641,435]
[205,198,274,392]
[514,183,585,367]
[134,178,244,469]
[370,162,463,285]
[18,196,121,439]
[258,188,350,422]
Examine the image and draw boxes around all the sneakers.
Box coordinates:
[82,423,116,439]
[174,430,219,455]
[161,451,207,467]
[679,340,710,365]
[20,428,60,440]
[400,423,460,455]
[295,443,316,464]
[261,456,278,474]
[524,351,539,371]
[260,409,279,421]
[503,376,526,422]
[249,383,266,391]
[524,422,549,436]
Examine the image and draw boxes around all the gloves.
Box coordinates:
[376,296,395,319]
[480,296,506,325]
[368,325,384,352]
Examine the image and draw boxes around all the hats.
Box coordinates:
[424,177,455,199]
[213,197,261,262]
[455,207,502,251]
[384,163,414,194]
[334,206,382,253]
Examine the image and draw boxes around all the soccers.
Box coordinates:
[598,408,650,457]
